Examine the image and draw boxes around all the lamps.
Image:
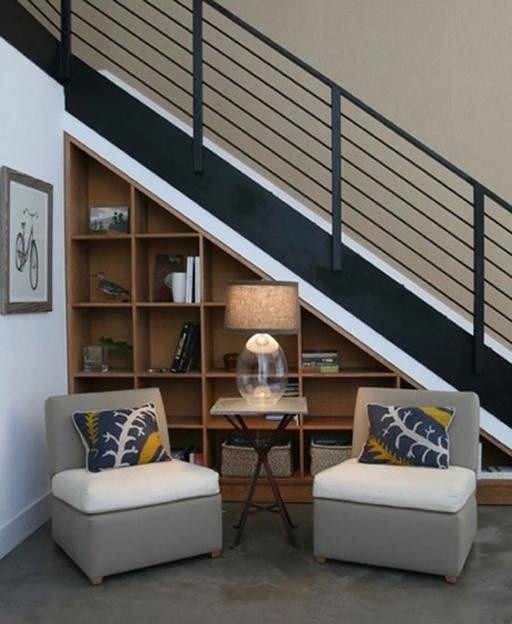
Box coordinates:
[222,281,301,407]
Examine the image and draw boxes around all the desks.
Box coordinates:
[210,396,308,549]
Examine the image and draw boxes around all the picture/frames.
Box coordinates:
[0,164,53,315]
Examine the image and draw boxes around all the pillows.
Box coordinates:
[69,401,172,474]
[357,402,456,469]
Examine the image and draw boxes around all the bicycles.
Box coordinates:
[14,211,39,291]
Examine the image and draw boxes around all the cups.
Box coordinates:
[82,346,109,374]
[171,271,186,304]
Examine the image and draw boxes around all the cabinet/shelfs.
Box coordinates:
[62,129,512,505]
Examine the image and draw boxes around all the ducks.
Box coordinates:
[91,271,130,297]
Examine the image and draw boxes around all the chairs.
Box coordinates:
[312,387,481,583]
[44,387,223,586]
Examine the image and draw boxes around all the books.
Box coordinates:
[185,255,194,303]
[263,349,341,421]
[481,465,511,480]
[170,320,200,373]
[193,255,201,303]
[184,444,204,466]
